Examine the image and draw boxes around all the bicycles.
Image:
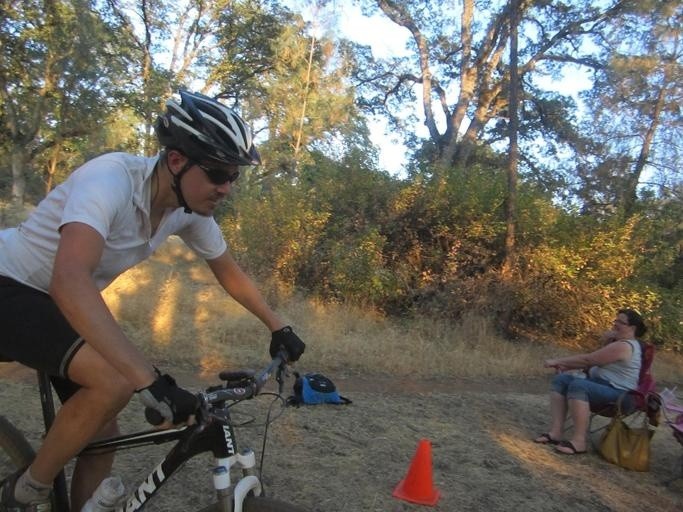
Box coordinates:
[0,344,309,512]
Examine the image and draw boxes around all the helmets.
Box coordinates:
[153,90,263,166]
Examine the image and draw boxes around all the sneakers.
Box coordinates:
[1,466,54,511]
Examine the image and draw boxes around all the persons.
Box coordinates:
[531,309,648,456]
[0,85,307,511]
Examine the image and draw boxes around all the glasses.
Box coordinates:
[196,161,240,185]
[613,318,630,327]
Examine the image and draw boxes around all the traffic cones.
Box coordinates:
[393,440,441,506]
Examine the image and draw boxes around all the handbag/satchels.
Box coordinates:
[597,417,655,473]
[294,372,341,404]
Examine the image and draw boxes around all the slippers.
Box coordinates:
[534,433,565,443]
[557,441,588,454]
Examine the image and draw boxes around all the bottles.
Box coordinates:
[79,475,126,511]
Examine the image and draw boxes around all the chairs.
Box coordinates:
[563,338,656,440]
